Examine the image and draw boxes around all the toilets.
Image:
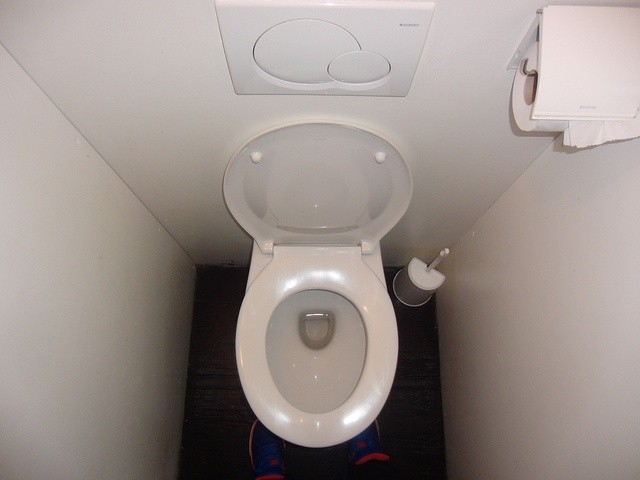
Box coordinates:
[212,112,415,450]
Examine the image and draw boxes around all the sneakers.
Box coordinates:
[348,419,389,466]
[249,420,287,479]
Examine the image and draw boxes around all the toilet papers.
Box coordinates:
[508,38,639,150]
